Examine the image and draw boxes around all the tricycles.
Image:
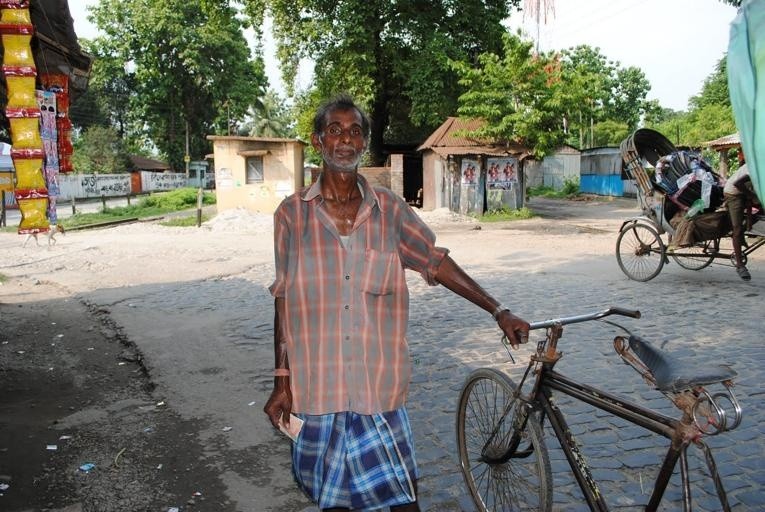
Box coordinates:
[615,128,765,282]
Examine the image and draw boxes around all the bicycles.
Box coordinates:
[455,306,742,512]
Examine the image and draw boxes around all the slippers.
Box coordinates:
[736,265,752,281]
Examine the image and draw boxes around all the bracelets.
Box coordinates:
[490,303,512,321]
[273,368,290,376]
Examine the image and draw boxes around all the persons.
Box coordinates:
[722,163,758,280]
[263,100,531,511]
